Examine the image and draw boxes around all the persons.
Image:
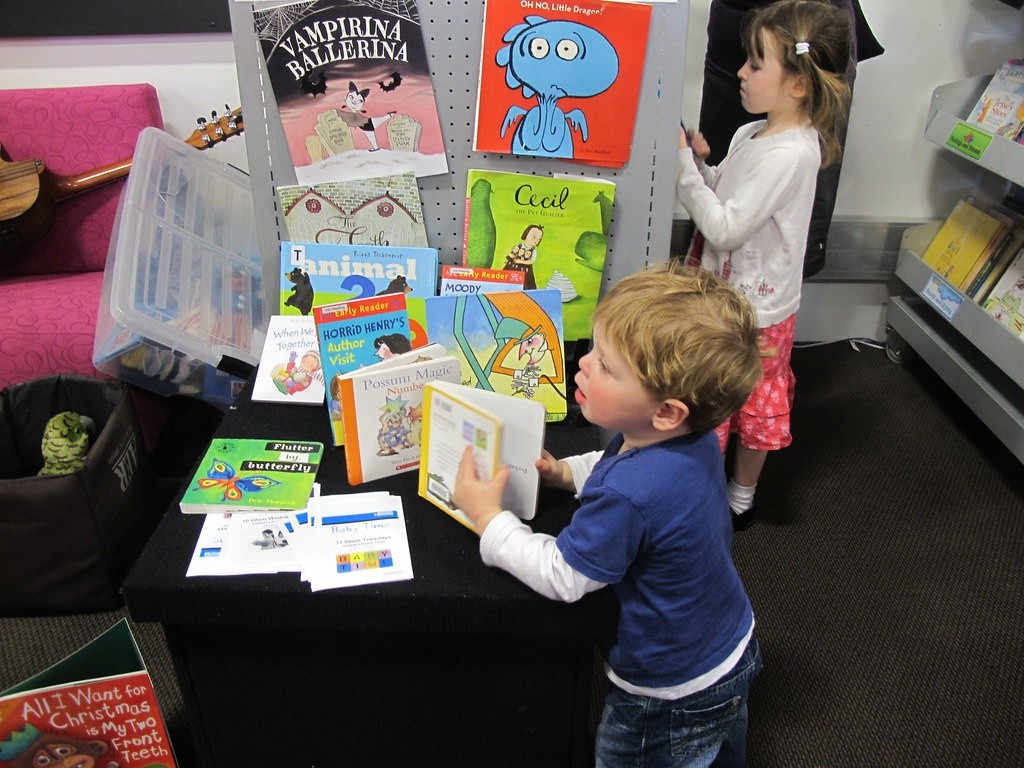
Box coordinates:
[453,269,764,768]
[682,1,886,278]
[674,0,853,532]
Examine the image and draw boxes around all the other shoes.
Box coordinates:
[728,497,757,531]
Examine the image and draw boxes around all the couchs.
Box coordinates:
[0,82,163,391]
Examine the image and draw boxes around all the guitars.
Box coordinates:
[0,104,245,259]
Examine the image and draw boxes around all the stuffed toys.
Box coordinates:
[35,410,96,478]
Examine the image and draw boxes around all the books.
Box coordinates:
[921,192,1024,343]
[312,292,414,447]
[439,266,526,297]
[177,438,324,515]
[460,169,616,342]
[418,381,549,539]
[274,172,433,247]
[279,241,441,316]
[965,57,1024,147]
[423,291,568,426]
[251,0,450,185]
[338,342,461,487]
[0,616,179,768]
[471,0,654,169]
[250,314,326,407]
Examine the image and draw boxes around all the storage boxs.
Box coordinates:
[91,126,266,407]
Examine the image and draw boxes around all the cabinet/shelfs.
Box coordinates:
[125,361,620,768]
[884,74,1024,464]
